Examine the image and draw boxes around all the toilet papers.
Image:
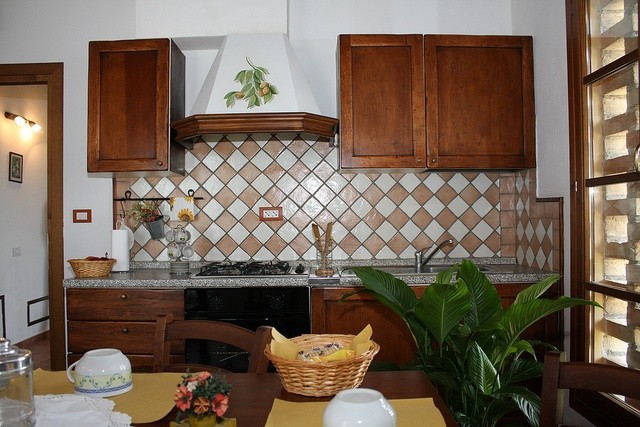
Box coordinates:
[112,229,129,271]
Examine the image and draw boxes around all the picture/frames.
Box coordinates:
[9,152,23,183]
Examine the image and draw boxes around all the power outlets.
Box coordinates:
[263,210,278,218]
[77,212,87,221]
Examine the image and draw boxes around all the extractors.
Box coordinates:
[177,111,339,149]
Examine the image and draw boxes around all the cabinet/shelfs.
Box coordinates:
[337,35,537,169]
[87,38,185,175]
[311,282,559,427]
[67,289,185,372]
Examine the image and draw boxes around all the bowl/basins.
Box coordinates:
[323,388,396,427]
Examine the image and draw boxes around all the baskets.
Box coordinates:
[263,334,380,397]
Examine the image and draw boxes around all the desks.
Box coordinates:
[0,369,463,427]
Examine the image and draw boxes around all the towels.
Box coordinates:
[170,197,194,222]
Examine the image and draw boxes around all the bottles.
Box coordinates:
[0,337,35,427]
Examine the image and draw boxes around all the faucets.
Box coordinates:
[414,239,455,272]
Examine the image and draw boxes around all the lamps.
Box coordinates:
[5,112,41,133]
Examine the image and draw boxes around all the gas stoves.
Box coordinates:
[200,261,310,283]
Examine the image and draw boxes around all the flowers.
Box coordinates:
[170,370,236,426]
[127,200,161,226]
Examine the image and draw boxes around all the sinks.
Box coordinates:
[371,265,490,276]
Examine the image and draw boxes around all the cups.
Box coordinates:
[67,348,133,398]
[165,227,194,262]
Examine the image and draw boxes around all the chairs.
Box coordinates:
[153,313,273,373]
[540,352,640,427]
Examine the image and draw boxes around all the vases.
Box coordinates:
[143,215,165,238]
